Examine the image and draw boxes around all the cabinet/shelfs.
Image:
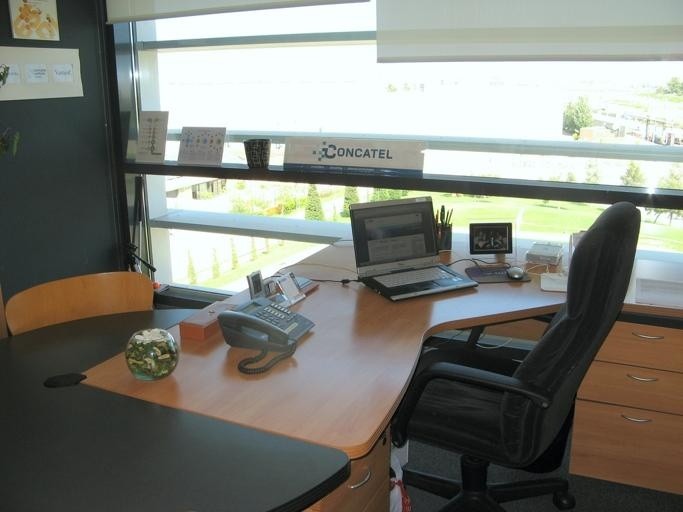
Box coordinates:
[123,154,683,307]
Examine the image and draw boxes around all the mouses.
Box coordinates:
[506,266,524,279]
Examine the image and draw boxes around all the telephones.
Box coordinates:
[217,297,315,352]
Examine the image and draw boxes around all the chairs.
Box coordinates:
[392,198,642,512]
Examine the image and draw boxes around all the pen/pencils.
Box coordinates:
[436,205,453,228]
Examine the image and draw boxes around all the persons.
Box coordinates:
[473,231,507,250]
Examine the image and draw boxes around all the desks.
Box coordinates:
[1,231,683,512]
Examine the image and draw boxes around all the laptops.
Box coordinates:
[349,196,479,301]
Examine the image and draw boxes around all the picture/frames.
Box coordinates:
[470,223,513,255]
